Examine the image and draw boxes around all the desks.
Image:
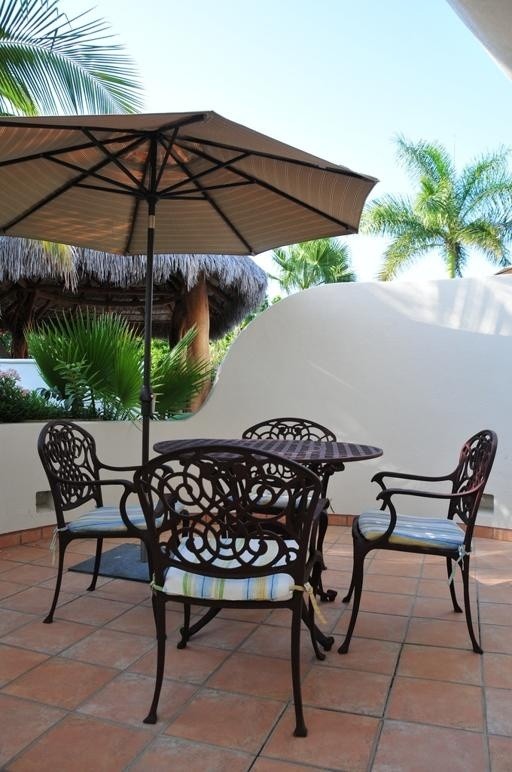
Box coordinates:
[153,438,383,653]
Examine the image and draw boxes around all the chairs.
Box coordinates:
[132,443,326,739]
[336,429,498,656]
[216,417,338,602]
[37,419,191,625]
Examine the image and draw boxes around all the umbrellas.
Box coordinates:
[0,110,382,583]
